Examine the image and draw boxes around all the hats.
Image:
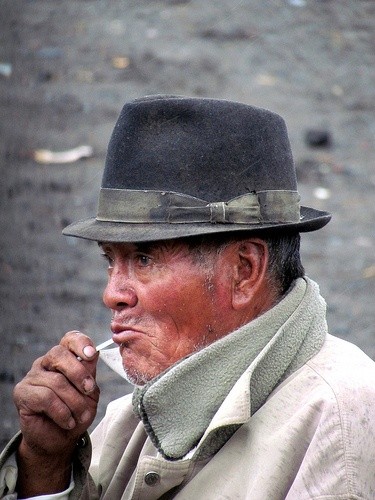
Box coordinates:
[57,93,332,243]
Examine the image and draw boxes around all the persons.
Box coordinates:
[0,96,375,500]
